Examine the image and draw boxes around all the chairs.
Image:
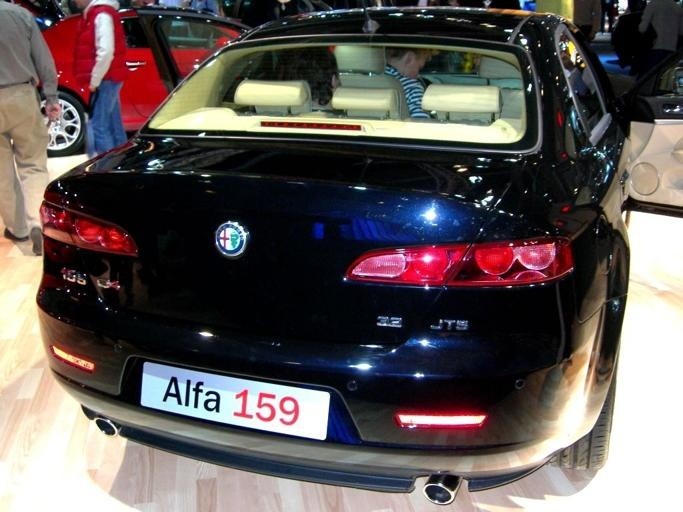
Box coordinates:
[334,45,410,121]
[480,56,523,119]
[332,88,401,120]
[422,84,503,124]
[234,79,312,116]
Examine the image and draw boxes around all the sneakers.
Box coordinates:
[3,227,43,256]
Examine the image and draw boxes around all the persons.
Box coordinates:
[366,48,433,118]
[0,1,62,256]
[0,0,683,73]
[74,0,128,156]
[284,48,349,118]
